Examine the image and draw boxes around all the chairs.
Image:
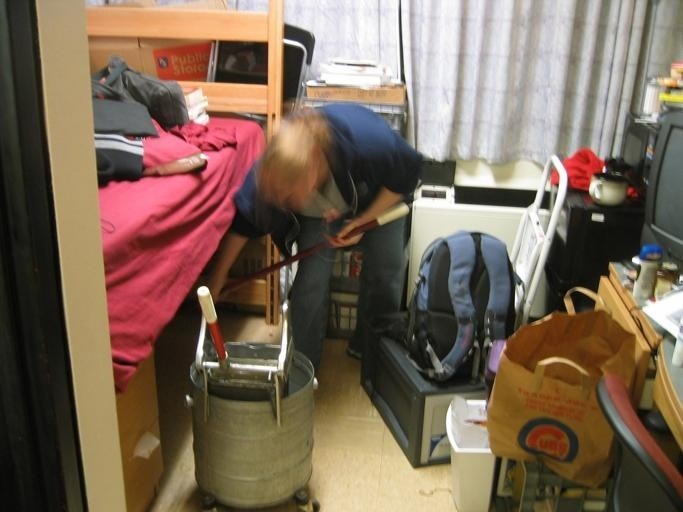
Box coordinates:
[591,376,683,512]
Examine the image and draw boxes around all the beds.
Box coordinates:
[83,0,289,510]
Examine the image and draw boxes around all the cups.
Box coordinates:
[588,171,628,207]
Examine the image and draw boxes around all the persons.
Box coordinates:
[200,97,426,387]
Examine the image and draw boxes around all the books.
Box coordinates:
[646,62,683,104]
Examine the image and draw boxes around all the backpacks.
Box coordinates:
[409,231,525,387]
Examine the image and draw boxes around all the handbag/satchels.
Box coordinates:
[486,285,639,491]
[90,59,128,101]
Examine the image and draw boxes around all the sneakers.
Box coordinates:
[345,333,364,357]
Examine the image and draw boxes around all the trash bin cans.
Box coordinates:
[445,399,495,512]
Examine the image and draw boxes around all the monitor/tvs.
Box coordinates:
[640,108,683,273]
